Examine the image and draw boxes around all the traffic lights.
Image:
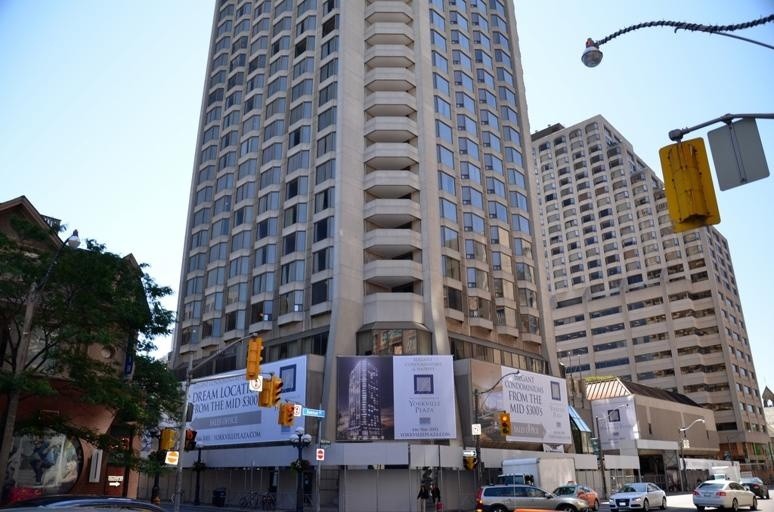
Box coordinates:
[284,403,295,426]
[462,455,474,470]
[500,413,513,437]
[184,429,198,452]
[272,375,283,407]
[246,337,265,379]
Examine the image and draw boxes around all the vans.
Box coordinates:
[475,482,590,512]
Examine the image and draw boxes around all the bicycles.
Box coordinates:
[240,489,276,512]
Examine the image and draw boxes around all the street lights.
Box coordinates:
[679,418,706,492]
[289,427,313,511]
[595,403,629,500]
[473,369,522,512]
[583,14,774,68]
[0,227,82,503]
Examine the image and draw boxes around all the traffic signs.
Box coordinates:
[303,407,325,418]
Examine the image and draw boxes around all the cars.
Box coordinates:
[549,485,600,512]
[607,482,668,511]
[0,492,171,512]
[692,479,758,512]
[740,477,771,499]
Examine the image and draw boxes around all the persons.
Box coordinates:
[417,485,429,512]
[431,482,440,512]
[666,477,702,492]
[19,435,61,486]
[1,445,18,506]
[57,460,78,496]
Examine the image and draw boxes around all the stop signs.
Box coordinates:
[317,450,324,460]
[166,452,177,463]
